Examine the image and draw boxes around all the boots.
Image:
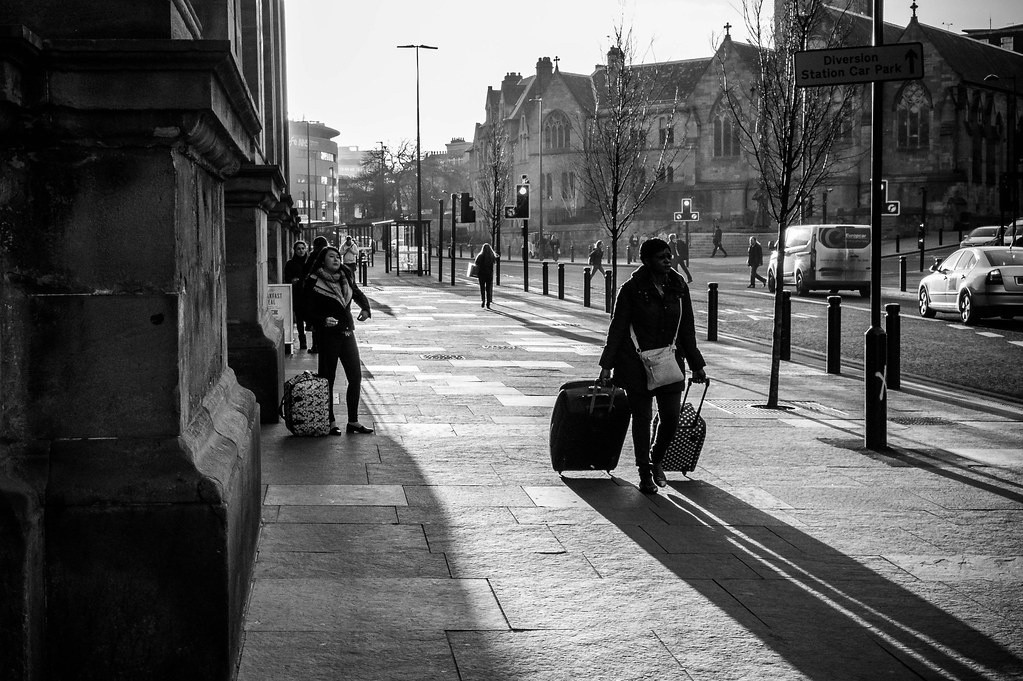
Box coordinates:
[298,335,307,349]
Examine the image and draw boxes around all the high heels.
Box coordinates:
[346,423,374,434]
[330,427,341,435]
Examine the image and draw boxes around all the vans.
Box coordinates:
[766,223,871,300]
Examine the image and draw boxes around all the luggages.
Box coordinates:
[649,377,710,476]
[549,378,631,475]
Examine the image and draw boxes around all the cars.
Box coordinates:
[917,239,1023,324]
[355,237,375,251]
[959,225,1003,250]
[519,231,560,258]
[1000,218,1023,247]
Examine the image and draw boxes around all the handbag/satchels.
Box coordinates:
[467,262,479,278]
[279,370,331,437]
[640,347,685,391]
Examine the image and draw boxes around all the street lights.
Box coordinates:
[375,140,385,221]
[397,43,440,271]
[301,120,313,224]
[529,97,546,263]
[328,166,336,225]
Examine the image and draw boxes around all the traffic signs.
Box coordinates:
[793,41,924,88]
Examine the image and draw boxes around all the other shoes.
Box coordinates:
[308,346,318,353]
[487,306,489,308]
[687,280,692,283]
[764,279,766,287]
[639,482,658,493]
[747,285,755,288]
[651,465,666,487]
[481,301,485,308]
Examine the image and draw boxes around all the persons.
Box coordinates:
[629,229,667,262]
[710,226,727,258]
[551,235,560,251]
[285,236,373,436]
[667,234,693,283]
[590,240,605,287]
[599,238,706,493]
[474,244,496,308]
[747,237,767,288]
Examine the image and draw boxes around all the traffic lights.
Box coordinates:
[879,178,899,215]
[503,182,530,219]
[673,198,701,223]
[461,191,477,224]
